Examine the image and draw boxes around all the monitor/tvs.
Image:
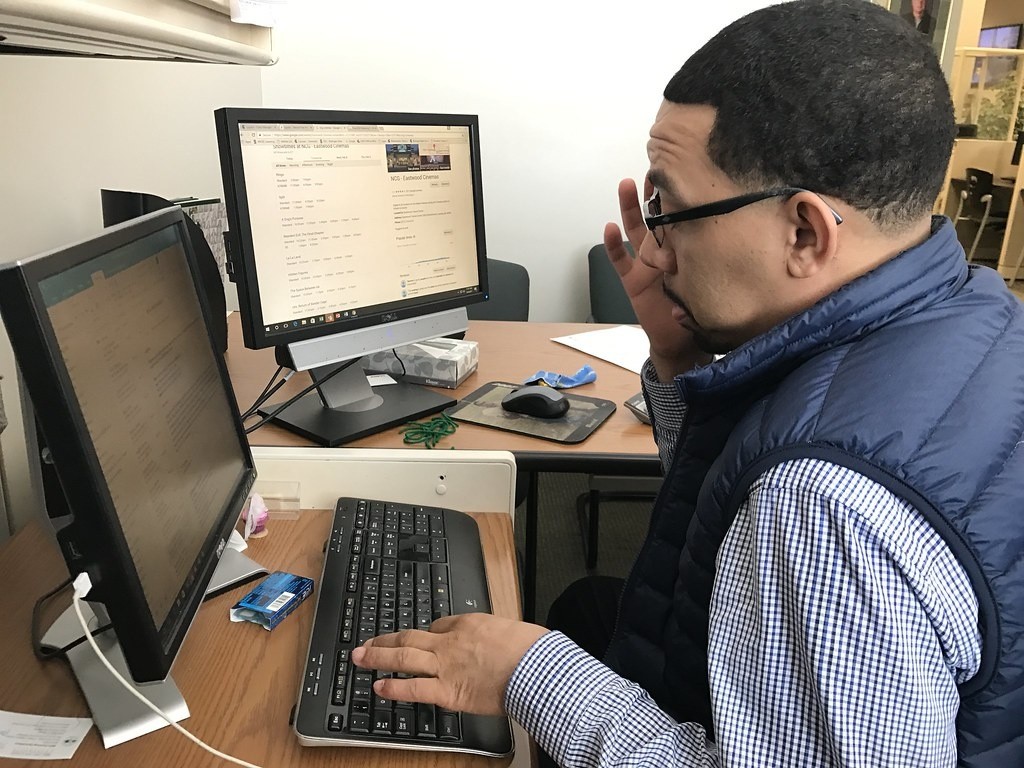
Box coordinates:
[214,107,490,447]
[0,204,267,750]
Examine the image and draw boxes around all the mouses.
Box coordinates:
[501,386,570,420]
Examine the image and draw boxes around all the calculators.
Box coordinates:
[625,393,652,425]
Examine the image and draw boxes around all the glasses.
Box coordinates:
[643,184,843,249]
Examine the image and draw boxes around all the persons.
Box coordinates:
[351,0,1024,768]
[900,0,935,35]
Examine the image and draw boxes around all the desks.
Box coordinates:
[0,322,667,768]
[932,139,1024,284]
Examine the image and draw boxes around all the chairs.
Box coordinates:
[576,238,662,571]
[952,167,1010,265]
[468,259,539,637]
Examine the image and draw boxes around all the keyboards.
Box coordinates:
[288,497,518,758]
